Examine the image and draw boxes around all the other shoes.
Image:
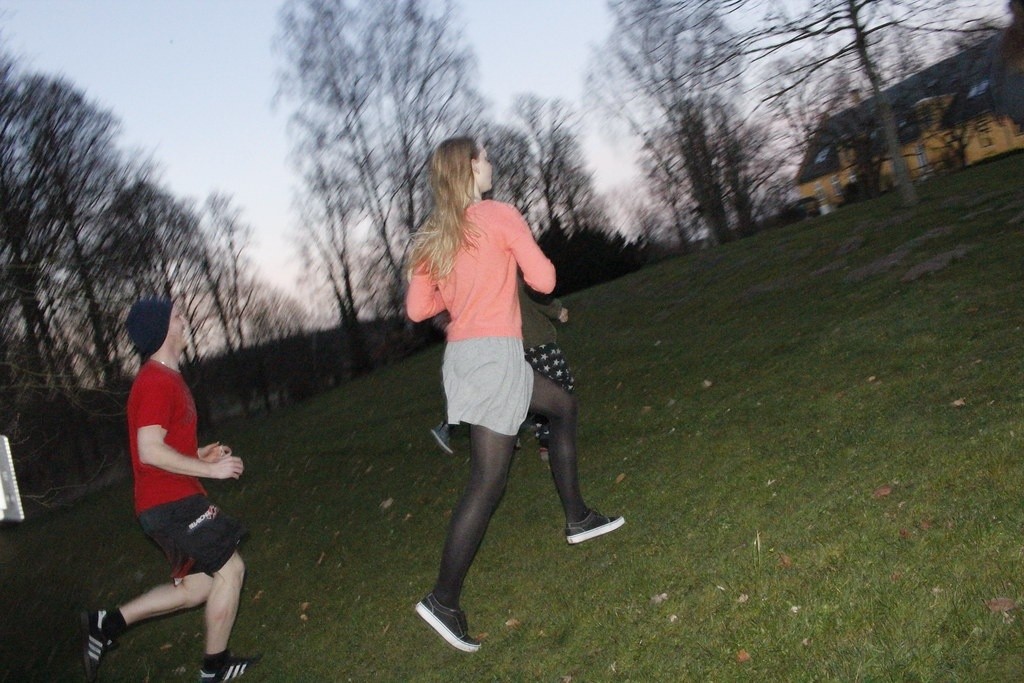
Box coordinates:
[538,448,548,463]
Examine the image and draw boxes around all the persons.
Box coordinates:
[407,136,627,653]
[80,299,262,683]
[517,273,575,461]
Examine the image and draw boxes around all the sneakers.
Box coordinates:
[515,436,522,450]
[430,420,455,457]
[415,592,482,652]
[564,508,626,544]
[79,609,119,683]
[198,652,265,683]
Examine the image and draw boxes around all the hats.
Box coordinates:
[125,295,175,357]
